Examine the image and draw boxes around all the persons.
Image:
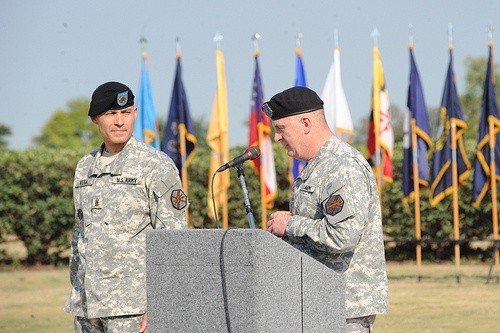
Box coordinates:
[262,86,389,333]
[62,82,188,333]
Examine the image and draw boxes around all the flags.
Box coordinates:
[367,49,395,188]
[473,41,500,206]
[288,45,358,198]
[248,51,276,209]
[400,45,440,203]
[428,45,471,205]
[208,47,229,221]
[138,55,198,196]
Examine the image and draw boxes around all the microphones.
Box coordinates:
[216,146,261,172]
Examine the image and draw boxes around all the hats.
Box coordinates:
[88,81,135,116]
[262,86,323,121]
